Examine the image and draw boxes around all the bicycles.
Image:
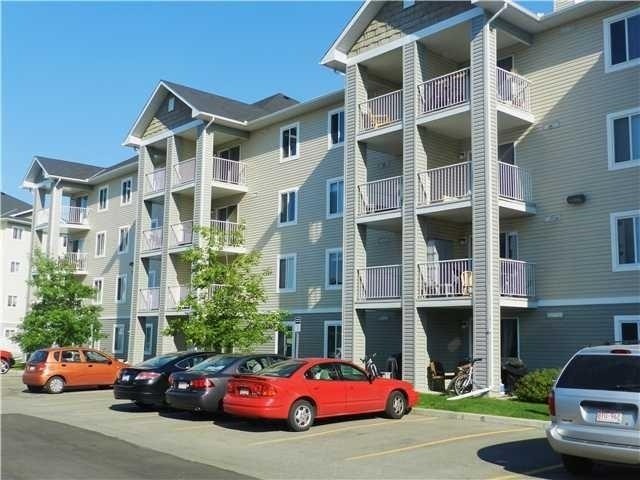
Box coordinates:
[446,358,482,397]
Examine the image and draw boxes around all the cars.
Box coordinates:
[0,350,17,376]
[545,340,639,473]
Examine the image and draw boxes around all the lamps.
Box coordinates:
[567,194,585,204]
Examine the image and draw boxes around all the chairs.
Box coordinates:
[313,368,333,380]
[250,363,262,374]
[431,361,445,392]
